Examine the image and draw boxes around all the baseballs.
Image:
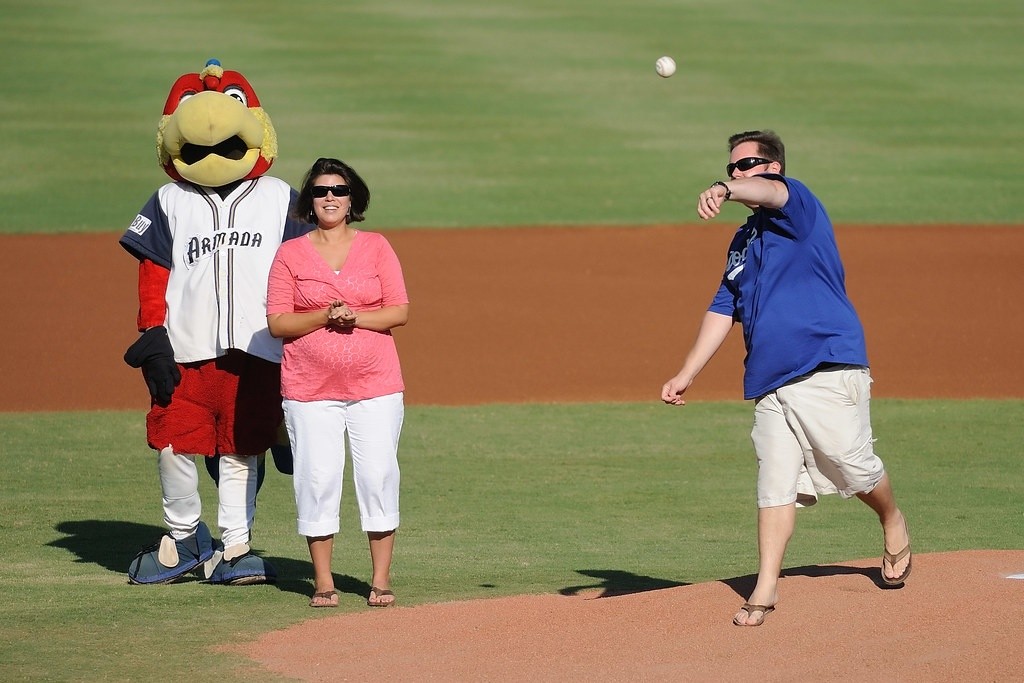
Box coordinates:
[655,57,677,78]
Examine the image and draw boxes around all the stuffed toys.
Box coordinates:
[124,59,318,582]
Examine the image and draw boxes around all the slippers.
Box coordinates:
[881,514,912,585]
[368,587,396,607]
[735,602,775,627]
[310,590,340,607]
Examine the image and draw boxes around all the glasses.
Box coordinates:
[310,185,351,198]
[726,157,772,177]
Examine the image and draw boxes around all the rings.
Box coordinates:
[706,197,713,201]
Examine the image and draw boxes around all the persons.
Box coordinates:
[266,159,408,605]
[662,129,912,623]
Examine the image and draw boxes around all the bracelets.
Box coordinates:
[710,181,730,201]
